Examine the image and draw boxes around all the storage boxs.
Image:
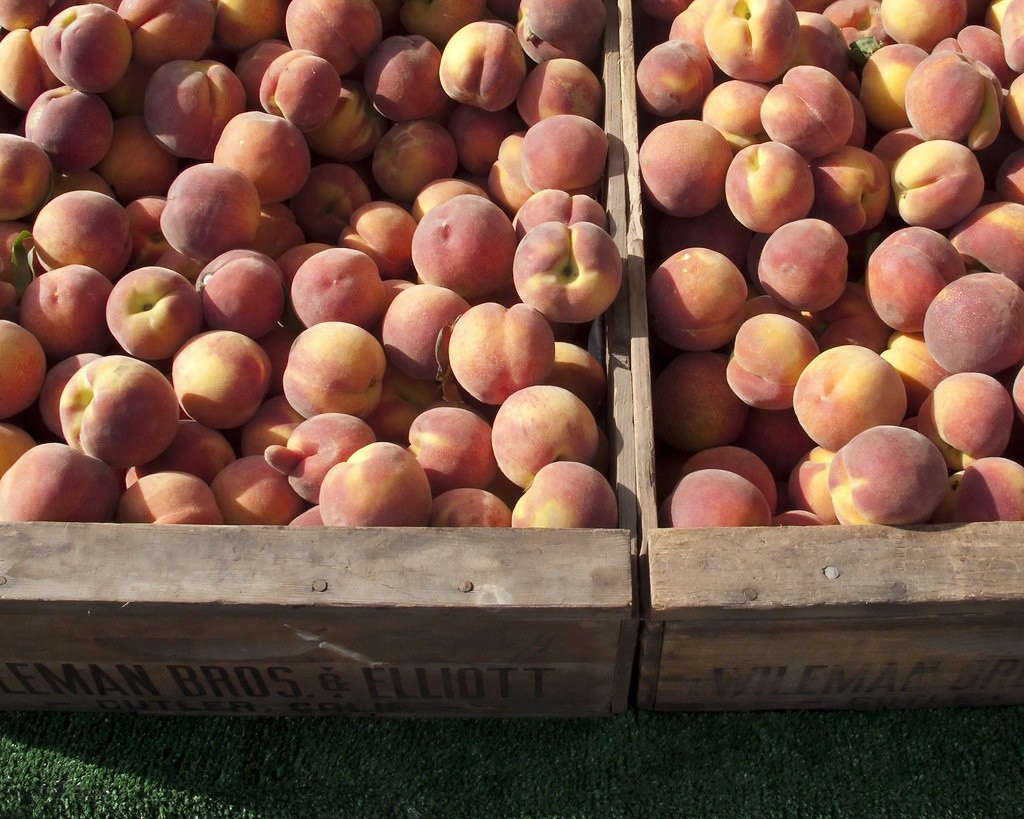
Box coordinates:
[1,0,1024,717]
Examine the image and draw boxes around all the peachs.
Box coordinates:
[0,0,1024,529]
